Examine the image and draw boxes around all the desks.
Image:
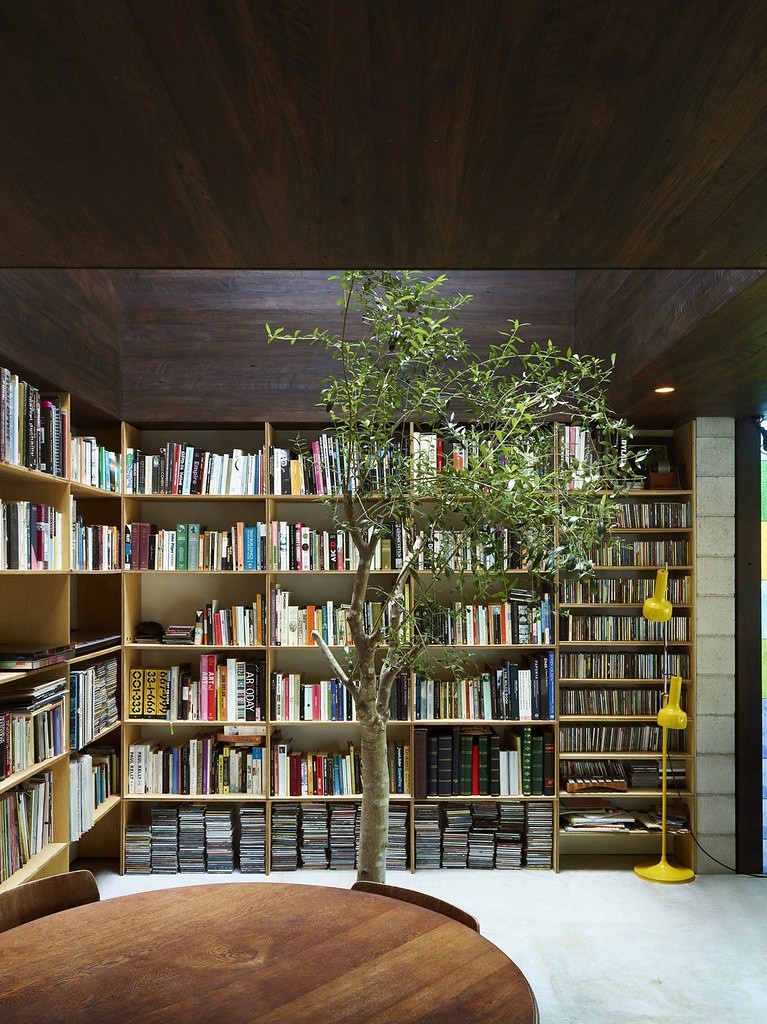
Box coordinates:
[0,882,541,1024]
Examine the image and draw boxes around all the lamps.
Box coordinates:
[642,567,673,694]
[633,671,694,884]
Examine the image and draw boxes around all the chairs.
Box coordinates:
[0,870,100,933]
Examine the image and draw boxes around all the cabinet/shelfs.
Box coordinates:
[122,420,698,877]
[0,392,122,894]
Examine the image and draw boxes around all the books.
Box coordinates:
[0,768,55,886]
[123,435,268,874]
[69,656,121,751]
[67,746,121,842]
[67,431,122,492]
[0,623,121,673]
[0,366,68,478]
[69,494,122,571]
[0,496,63,571]
[0,674,68,784]
[270,425,413,872]
[553,421,696,873]
[396,427,555,871]
